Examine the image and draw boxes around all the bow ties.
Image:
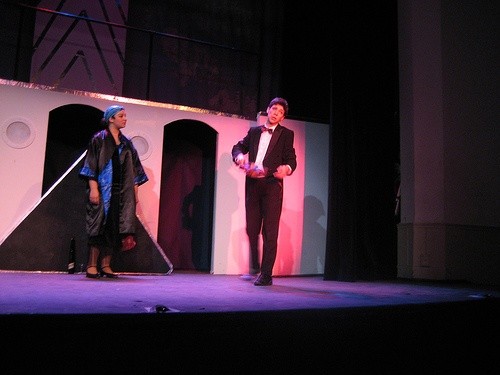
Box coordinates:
[260,125,274,134]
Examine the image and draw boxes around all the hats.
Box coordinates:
[103,105,124,123]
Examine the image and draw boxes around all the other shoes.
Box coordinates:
[86,266,99,278]
[256,272,273,285]
[249,261,259,273]
[100,266,118,278]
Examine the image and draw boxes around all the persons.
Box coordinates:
[232,97,297,285]
[80,105,149,278]
